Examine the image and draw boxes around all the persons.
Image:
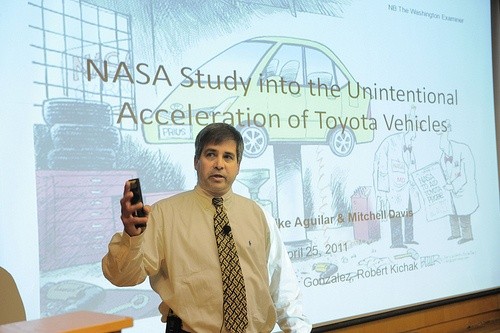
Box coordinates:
[102,122,314,333]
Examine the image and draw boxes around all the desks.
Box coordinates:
[0,310,133,333]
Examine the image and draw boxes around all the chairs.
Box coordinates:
[0,267,26,325]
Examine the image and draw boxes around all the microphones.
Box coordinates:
[222,225,232,236]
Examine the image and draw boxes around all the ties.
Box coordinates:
[212,196,248,333]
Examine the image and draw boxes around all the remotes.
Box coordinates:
[129,178,145,227]
